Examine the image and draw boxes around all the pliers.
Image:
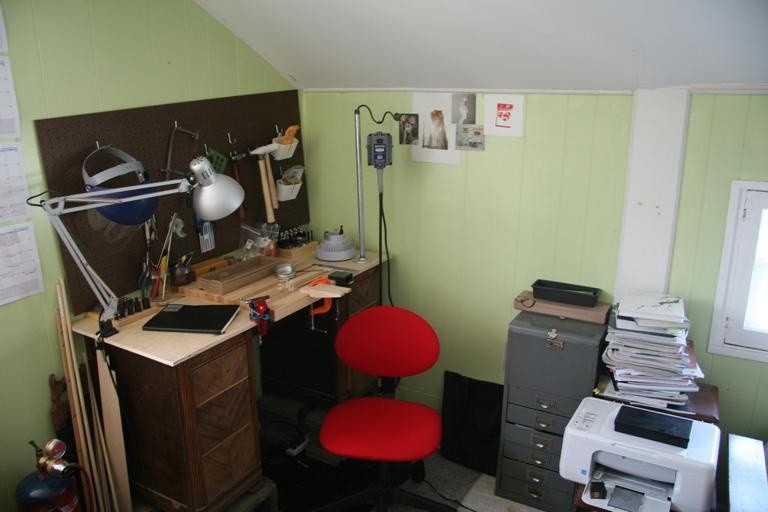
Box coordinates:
[138,260,161,300]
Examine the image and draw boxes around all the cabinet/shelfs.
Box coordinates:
[84,337,260,511]
[495,308,609,512]
[261,263,382,405]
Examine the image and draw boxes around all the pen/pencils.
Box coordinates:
[276,224,313,249]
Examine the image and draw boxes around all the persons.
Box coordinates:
[398,95,481,148]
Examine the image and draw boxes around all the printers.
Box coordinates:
[558,396,721,512]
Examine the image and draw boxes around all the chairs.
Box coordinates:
[319,306,445,505]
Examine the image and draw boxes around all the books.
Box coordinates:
[601,290,704,415]
[143,302,242,335]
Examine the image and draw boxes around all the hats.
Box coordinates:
[81,145,158,225]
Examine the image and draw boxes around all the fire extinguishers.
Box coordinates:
[15,439,87,512]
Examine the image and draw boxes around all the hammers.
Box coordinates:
[250,143,280,224]
[231,153,246,219]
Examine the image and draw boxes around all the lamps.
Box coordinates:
[40,156,246,340]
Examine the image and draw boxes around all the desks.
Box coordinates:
[67,236,395,511]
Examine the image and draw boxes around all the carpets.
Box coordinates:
[457,468,549,511]
